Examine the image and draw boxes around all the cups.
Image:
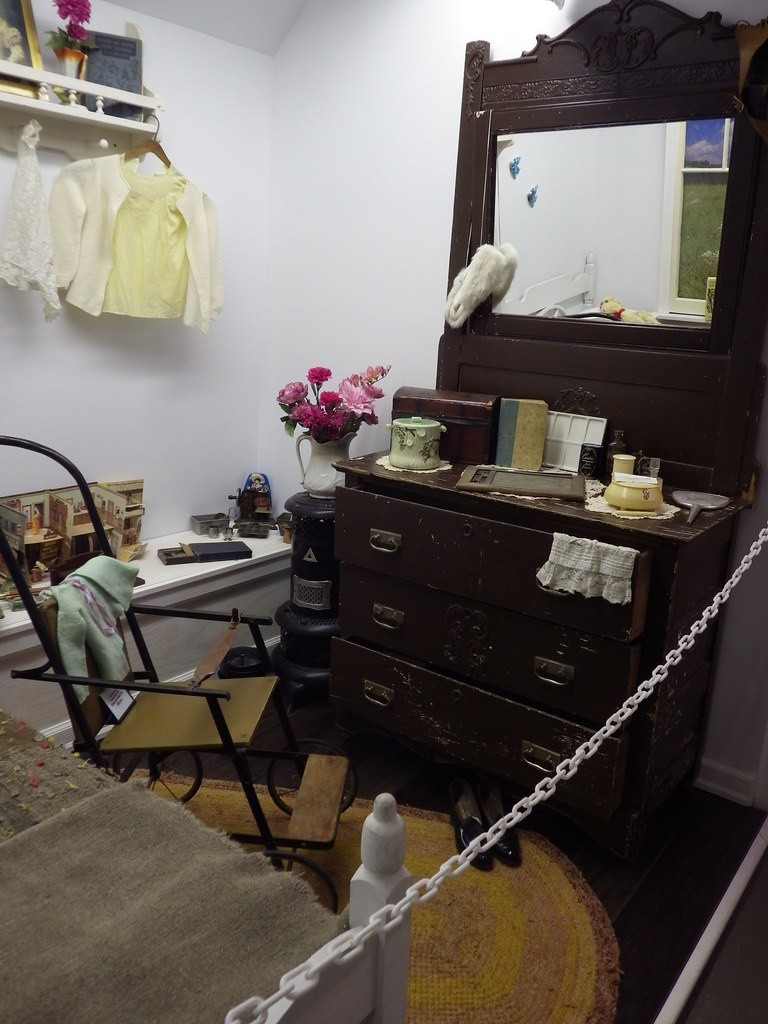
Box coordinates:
[385,415,448,471]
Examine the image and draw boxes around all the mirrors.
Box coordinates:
[465,109,742,345]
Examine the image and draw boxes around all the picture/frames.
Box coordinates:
[0,0,48,101]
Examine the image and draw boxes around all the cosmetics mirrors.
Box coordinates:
[673,488,732,525]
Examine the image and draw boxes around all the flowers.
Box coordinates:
[51,0,95,52]
[277,362,391,441]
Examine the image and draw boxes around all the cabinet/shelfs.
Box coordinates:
[308,1,767,864]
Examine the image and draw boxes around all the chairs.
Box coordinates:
[0,431,368,917]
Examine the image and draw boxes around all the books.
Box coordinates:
[494,396,550,473]
[82,28,146,126]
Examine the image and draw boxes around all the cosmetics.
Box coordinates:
[577,428,665,517]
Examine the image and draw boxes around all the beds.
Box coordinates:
[0,704,415,1024]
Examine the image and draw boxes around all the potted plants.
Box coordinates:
[55,43,86,82]
[293,431,357,498]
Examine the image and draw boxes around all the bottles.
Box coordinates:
[601,473,662,513]
[612,454,638,476]
[577,442,604,481]
[604,429,628,486]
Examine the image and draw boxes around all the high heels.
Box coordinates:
[481,784,521,867]
[450,787,494,871]
